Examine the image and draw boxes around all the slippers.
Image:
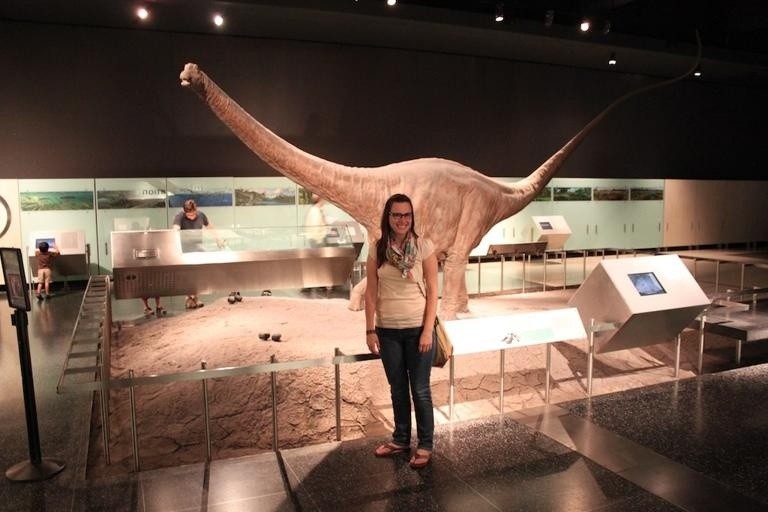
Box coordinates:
[374,443,410,456]
[410,452,432,467]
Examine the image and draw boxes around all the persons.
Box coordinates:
[172,200,222,309]
[365,194,439,467]
[307,193,338,246]
[35,242,59,300]
[141,296,165,316]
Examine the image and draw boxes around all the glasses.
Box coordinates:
[392,212,411,218]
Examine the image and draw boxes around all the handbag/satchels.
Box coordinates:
[432,316,452,368]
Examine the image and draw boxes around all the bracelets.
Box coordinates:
[366,329,376,334]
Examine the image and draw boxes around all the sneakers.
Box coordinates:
[185,295,203,308]
[156,307,166,313]
[144,308,154,314]
[36,295,50,300]
